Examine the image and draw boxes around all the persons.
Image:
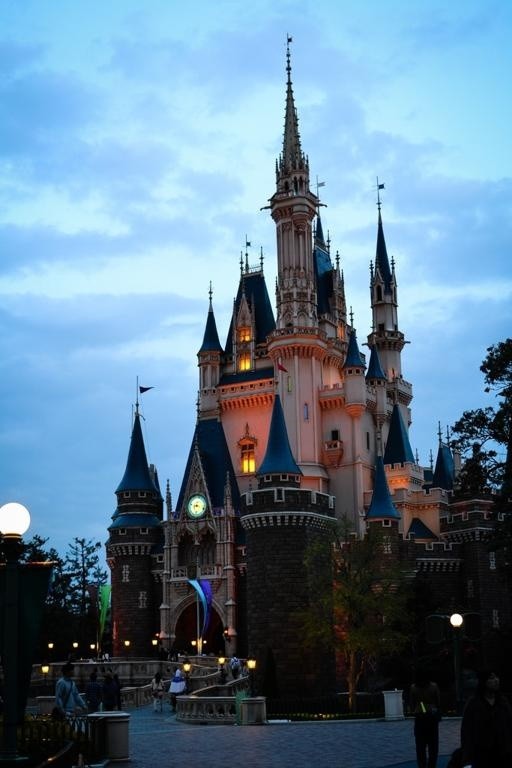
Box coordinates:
[231,653,240,680]
[160,647,179,662]
[150,672,164,712]
[411,664,442,766]
[461,668,512,767]
[102,650,109,662]
[170,668,187,712]
[55,663,89,718]
[217,655,227,683]
[85,672,122,714]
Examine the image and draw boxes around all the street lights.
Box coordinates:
[0,501,31,766]
[151,638,158,657]
[183,657,192,694]
[40,662,50,686]
[124,640,130,660]
[218,656,225,685]
[449,613,464,715]
[47,640,96,656]
[246,655,257,697]
[190,639,197,655]
